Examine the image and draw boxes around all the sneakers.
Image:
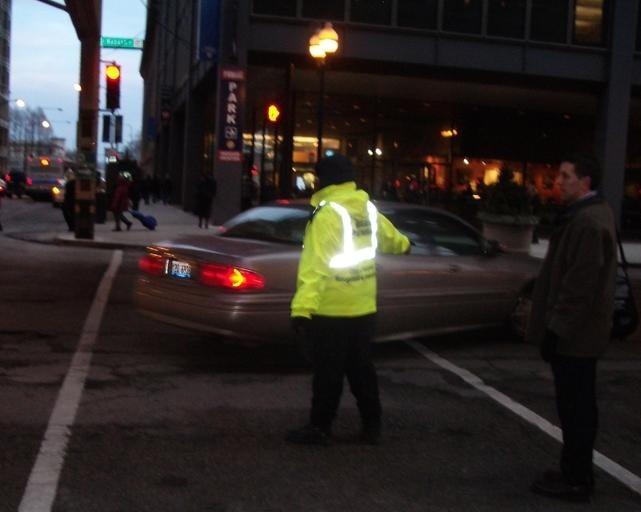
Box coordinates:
[286,424,333,445]
[340,423,379,442]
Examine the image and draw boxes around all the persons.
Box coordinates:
[63,170,218,232]
[288,147,411,446]
[527,145,618,501]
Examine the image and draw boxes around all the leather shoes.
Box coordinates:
[530,463,594,501]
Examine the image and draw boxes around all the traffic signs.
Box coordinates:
[101,36,144,49]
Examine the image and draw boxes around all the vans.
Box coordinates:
[23,153,77,198]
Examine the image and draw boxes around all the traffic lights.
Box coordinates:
[262,100,281,128]
[103,62,124,111]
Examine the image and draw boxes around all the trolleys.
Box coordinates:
[125,208,157,231]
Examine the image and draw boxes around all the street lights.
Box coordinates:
[306,21,341,168]
[1,98,27,108]
[50,118,73,126]
[21,105,63,181]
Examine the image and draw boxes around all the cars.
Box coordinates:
[0,168,24,199]
[517,193,641,267]
[127,194,545,364]
